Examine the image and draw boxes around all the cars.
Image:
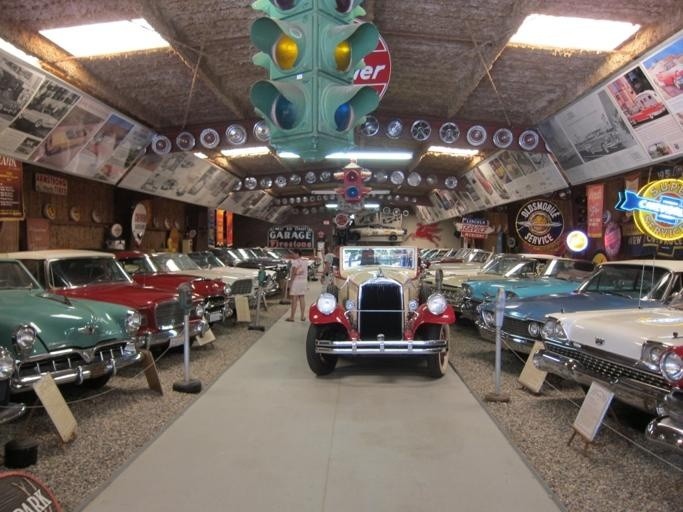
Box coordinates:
[348,223,408,241]
[655,60,682,91]
[579,128,622,155]
[518,150,543,164]
[20,109,57,129]
[0,88,18,115]
[626,90,667,124]
[44,126,88,155]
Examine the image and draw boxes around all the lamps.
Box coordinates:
[364,204,380,210]
[278,152,299,161]
[324,150,416,162]
[324,202,338,209]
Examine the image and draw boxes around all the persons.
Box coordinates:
[324,248,335,269]
[284,249,309,322]
